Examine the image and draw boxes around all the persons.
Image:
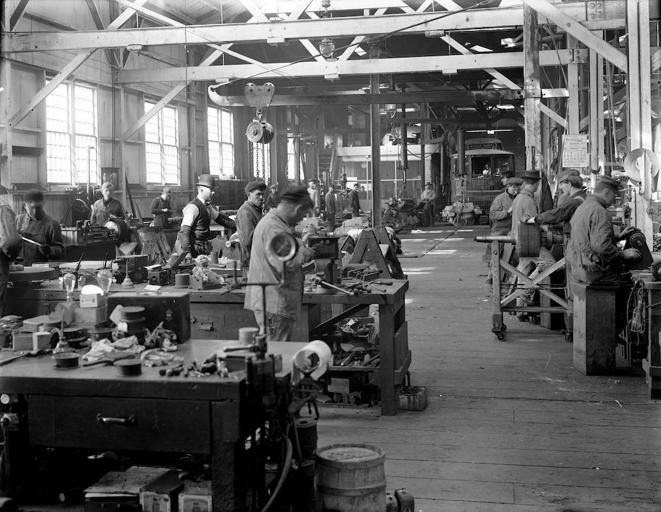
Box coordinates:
[244,187,310,342]
[1,196,22,298]
[151,174,361,265]
[421,182,437,225]
[519,168,591,342]
[571,175,642,346]
[15,191,66,262]
[501,170,511,185]
[90,182,126,223]
[512,170,556,323]
[483,178,523,268]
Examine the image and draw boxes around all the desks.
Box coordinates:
[0,218,410,512]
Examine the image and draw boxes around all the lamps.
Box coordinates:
[318,0,335,58]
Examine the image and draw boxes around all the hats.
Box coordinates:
[194,174,314,201]
[507,169,621,196]
